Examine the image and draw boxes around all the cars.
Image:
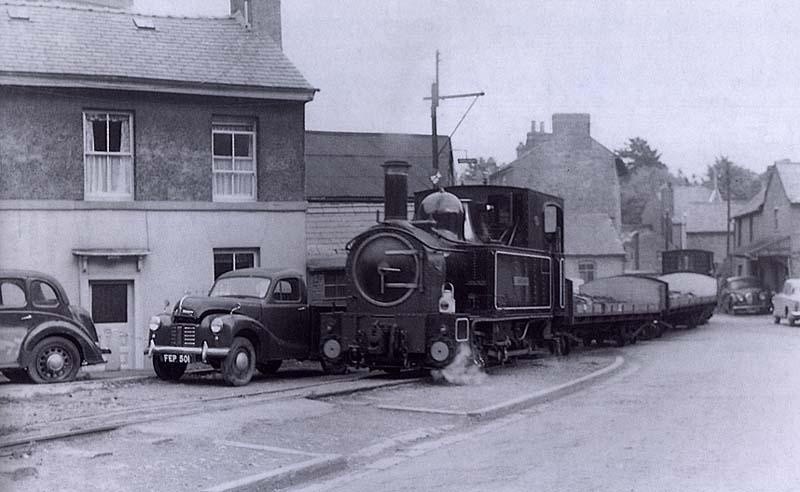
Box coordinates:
[141,268,314,386]
[721,276,800,326]
[0,269,111,383]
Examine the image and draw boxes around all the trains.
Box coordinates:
[318,185,718,375]
